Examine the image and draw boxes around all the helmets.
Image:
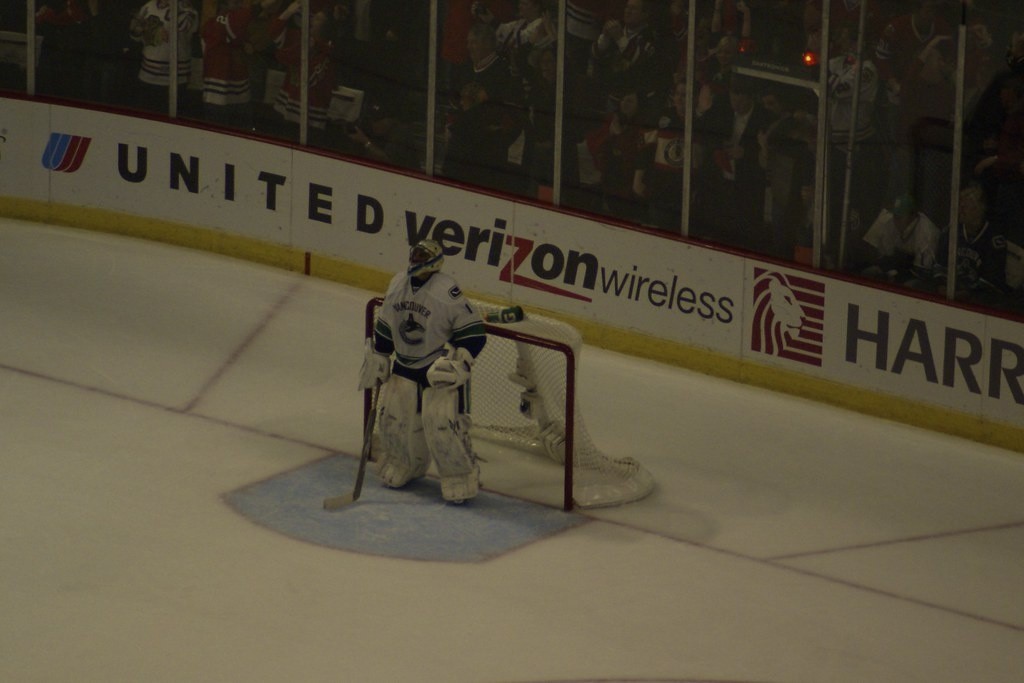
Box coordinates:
[406,238,446,281]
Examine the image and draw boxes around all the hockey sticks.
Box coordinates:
[323,376,381,513]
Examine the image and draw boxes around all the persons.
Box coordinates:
[0,0,1024,322]
[358,240,487,503]
